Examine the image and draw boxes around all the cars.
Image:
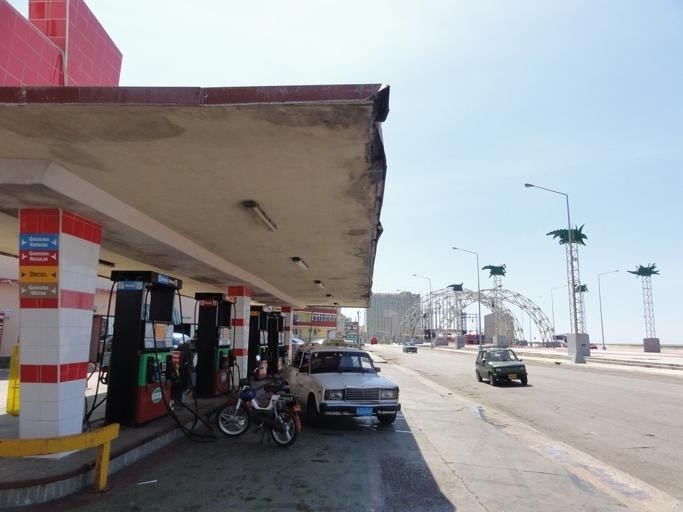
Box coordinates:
[475,348,528,386]
[284,346,401,428]
[403,342,417,353]
[590,345,597,349]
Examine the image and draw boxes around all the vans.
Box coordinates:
[552,335,567,348]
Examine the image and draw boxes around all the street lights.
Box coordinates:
[524,183,585,363]
[528,296,543,347]
[413,274,434,349]
[597,269,620,351]
[453,246,482,346]
[550,283,568,343]
[482,285,502,299]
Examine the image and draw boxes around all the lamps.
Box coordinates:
[291,256,308,271]
[313,280,339,307]
[241,200,276,233]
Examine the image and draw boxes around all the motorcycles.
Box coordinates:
[216,354,303,448]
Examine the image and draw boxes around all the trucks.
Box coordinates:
[466,334,484,344]
